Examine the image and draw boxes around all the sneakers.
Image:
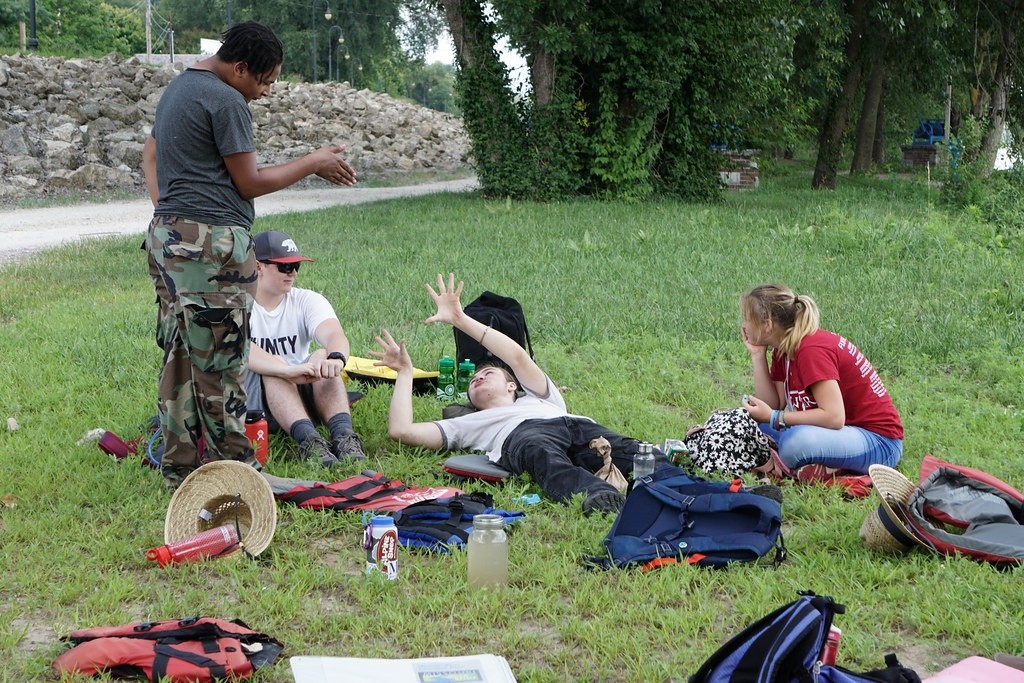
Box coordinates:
[330,427,367,461]
[298,432,338,468]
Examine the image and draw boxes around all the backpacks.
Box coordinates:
[687,590,922,683]
[452,290,537,393]
[576,465,787,571]
[390,491,526,555]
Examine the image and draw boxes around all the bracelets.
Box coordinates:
[770,410,786,431]
[480,326,489,344]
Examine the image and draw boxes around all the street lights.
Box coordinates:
[336,44,351,82]
[328,24,345,83]
[349,58,363,88]
[311,0,334,84]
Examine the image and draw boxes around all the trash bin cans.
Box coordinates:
[914,124,959,174]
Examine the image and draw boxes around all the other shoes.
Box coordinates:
[797,464,854,487]
[582,489,625,519]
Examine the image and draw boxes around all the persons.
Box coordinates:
[242,228,366,469]
[738,282,905,486]
[140,21,358,488]
[369,272,785,519]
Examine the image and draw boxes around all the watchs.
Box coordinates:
[327,351,346,368]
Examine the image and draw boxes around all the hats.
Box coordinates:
[858,463,947,558]
[253,230,315,263]
[163,460,277,559]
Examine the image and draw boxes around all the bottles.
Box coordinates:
[468,514,508,591]
[632,440,655,480]
[457,361,475,402]
[820,624,842,667]
[245,409,267,465]
[438,355,454,402]
[146,523,238,567]
[366,513,397,580]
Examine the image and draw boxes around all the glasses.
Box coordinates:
[257,259,301,273]
